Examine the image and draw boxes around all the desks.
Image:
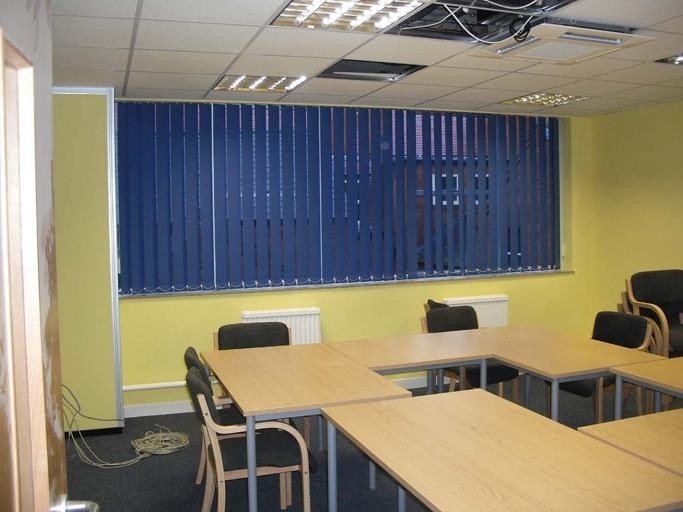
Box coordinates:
[321,321,683,492]
[318,384,683,512]
[203,342,413,512]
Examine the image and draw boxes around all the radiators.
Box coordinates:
[443,294,509,327]
[242,307,321,344]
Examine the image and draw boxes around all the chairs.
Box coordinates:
[184,346,318,512]
[420,306,519,406]
[545,269,683,424]
